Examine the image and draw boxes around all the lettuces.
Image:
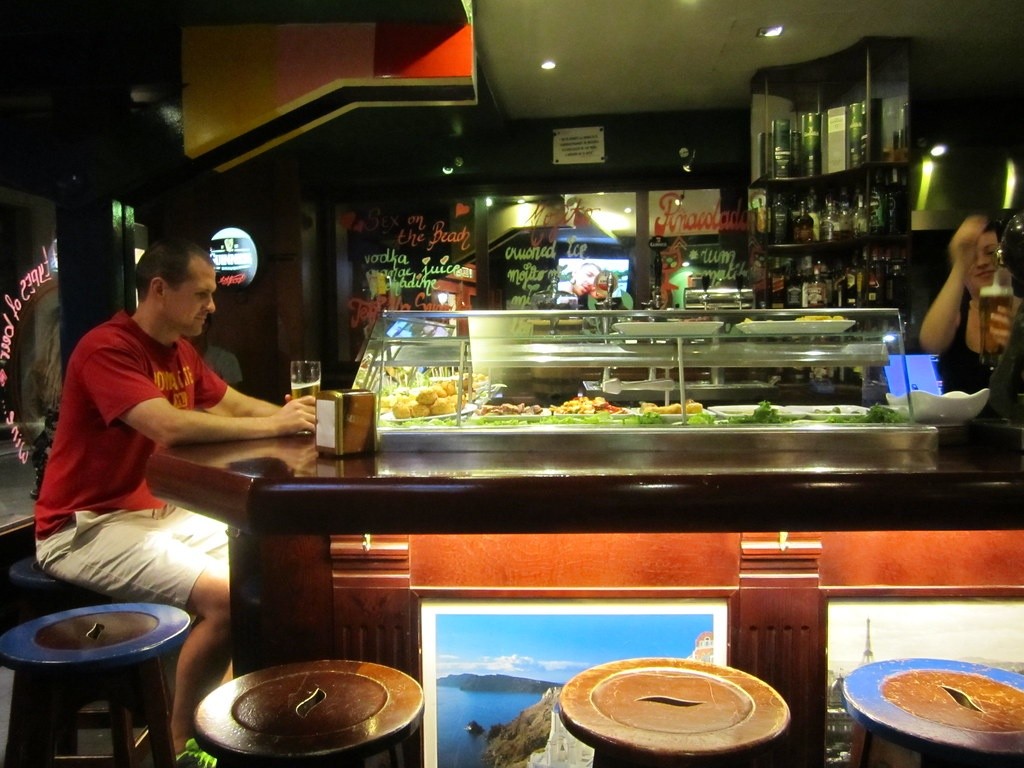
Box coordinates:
[378,400,912,429]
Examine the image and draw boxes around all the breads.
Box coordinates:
[392,382,467,418]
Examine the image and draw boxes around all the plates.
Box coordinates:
[379,403,477,422]
[472,405,871,419]
[735,320,856,334]
[611,322,724,335]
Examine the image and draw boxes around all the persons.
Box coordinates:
[530,263,600,399]
[920,212,1024,419]
[34,241,315,768]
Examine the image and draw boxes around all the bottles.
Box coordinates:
[754,168,908,308]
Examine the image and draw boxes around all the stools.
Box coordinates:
[556,658,791,768]
[189,658,425,768]
[7,556,152,731]
[0,603,192,768]
[839,656,1024,768]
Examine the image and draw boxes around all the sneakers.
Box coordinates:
[174,738,218,768]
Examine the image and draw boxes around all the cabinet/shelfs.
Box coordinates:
[744,34,919,335]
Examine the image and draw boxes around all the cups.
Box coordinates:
[979,286,1014,365]
[290,359,321,434]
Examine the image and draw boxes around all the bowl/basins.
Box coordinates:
[885,387,991,427]
